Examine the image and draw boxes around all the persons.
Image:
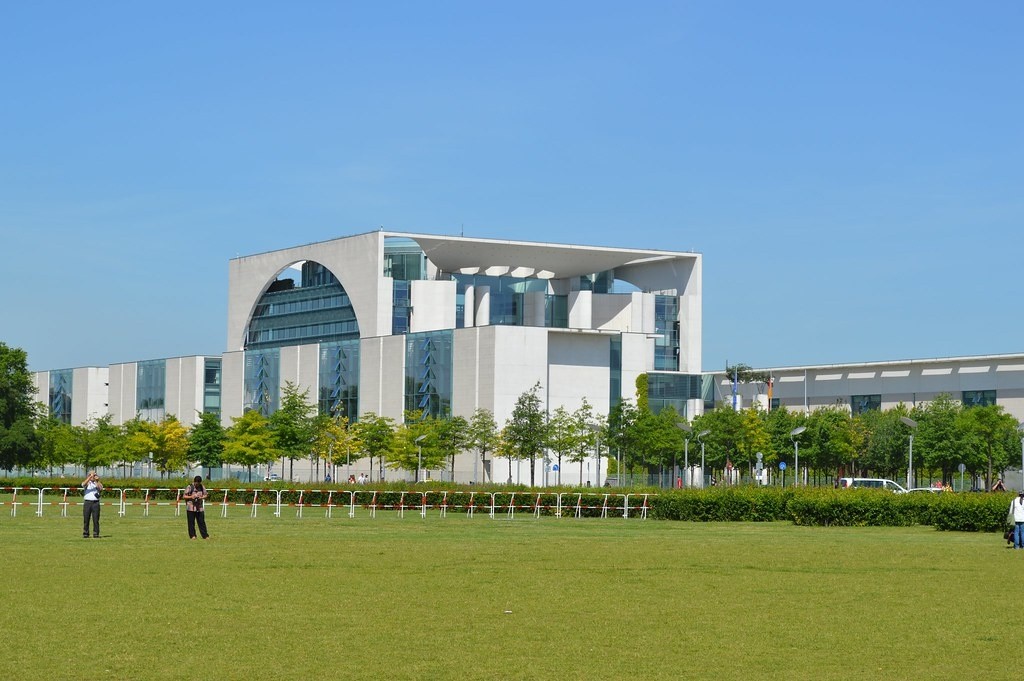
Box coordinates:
[677,476,684,489]
[929,478,955,493]
[1005,527,1016,545]
[711,477,718,485]
[81,471,103,539]
[992,479,1007,490]
[348,471,370,483]
[183,476,210,540]
[1008,486,1024,549]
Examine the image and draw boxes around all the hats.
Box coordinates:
[194,476,202,483]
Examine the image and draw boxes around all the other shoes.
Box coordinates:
[84,534,100,538]
[191,535,210,539]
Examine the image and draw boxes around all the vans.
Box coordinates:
[835,478,908,496]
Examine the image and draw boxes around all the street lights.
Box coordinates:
[901,416,918,493]
[586,424,602,489]
[677,423,692,488]
[697,430,711,489]
[416,434,427,483]
[1017,422,1024,495]
[790,427,806,488]
